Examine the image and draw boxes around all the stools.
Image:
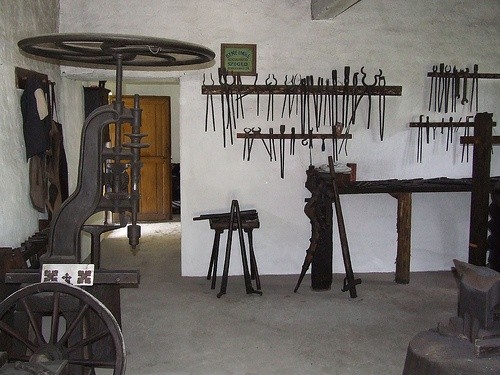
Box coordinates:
[206,213,262,291]
